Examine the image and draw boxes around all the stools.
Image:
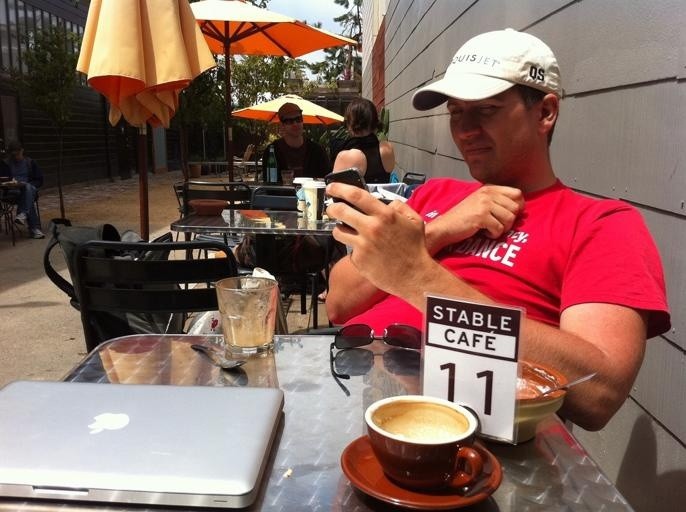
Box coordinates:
[0,192,43,248]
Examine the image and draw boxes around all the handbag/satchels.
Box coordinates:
[44,219,184,354]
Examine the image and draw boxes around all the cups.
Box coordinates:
[302,180,329,221]
[215,276,279,353]
[364,394,483,490]
[293,177,317,218]
[281,170,295,186]
[511,357,571,445]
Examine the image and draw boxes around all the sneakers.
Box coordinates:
[29,227,45,239]
[15,214,26,226]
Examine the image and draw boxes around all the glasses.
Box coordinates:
[330,324,423,380]
[284,116,302,124]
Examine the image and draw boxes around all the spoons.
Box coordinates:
[191,365,248,387]
[191,345,247,371]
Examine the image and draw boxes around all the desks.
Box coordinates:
[0,330,637,512]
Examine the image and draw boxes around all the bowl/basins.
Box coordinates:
[189,199,226,218]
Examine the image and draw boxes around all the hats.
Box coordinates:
[10,140,22,154]
[411,28,563,111]
[278,102,303,121]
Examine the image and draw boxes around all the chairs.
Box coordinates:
[169,168,430,330]
[68,240,241,360]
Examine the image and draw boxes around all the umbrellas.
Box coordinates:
[231,93,345,125]
[76,0,217,242]
[187,0,358,236]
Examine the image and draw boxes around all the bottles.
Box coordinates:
[266,146,278,184]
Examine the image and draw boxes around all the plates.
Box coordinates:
[342,433,501,511]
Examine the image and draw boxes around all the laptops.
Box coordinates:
[0,380,284,508]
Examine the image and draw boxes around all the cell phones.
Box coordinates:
[325,168,370,232]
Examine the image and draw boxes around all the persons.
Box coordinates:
[365,339,424,395]
[317,97,395,301]
[324,27,672,430]
[0,141,46,239]
[263,103,331,196]
